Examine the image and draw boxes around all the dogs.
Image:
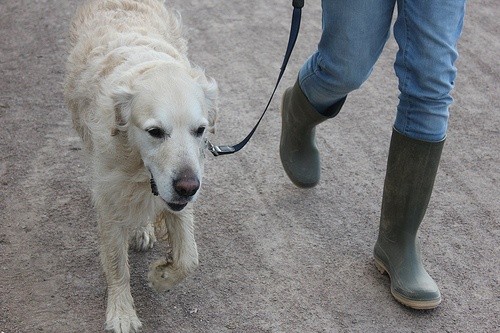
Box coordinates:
[64,0,220,333]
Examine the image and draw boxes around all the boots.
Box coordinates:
[372,125,447,309]
[280,72,348,189]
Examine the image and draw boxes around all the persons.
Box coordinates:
[279,0,464,310]
